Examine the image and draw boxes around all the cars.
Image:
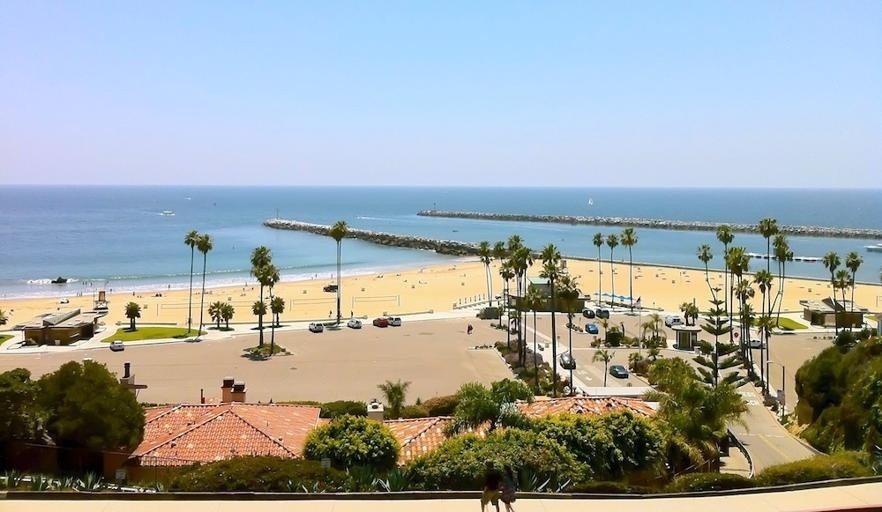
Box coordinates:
[744,339,766,349]
[110,340,124,351]
[586,324,598,334]
[560,352,576,368]
[347,319,361,329]
[583,309,609,319]
[373,317,401,327]
[610,364,629,378]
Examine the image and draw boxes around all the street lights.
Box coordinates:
[767,360,785,418]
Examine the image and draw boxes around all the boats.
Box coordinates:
[52,277,67,284]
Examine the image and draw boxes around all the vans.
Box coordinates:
[309,322,323,333]
[665,316,681,328]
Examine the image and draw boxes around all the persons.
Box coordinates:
[481,458,516,512]
